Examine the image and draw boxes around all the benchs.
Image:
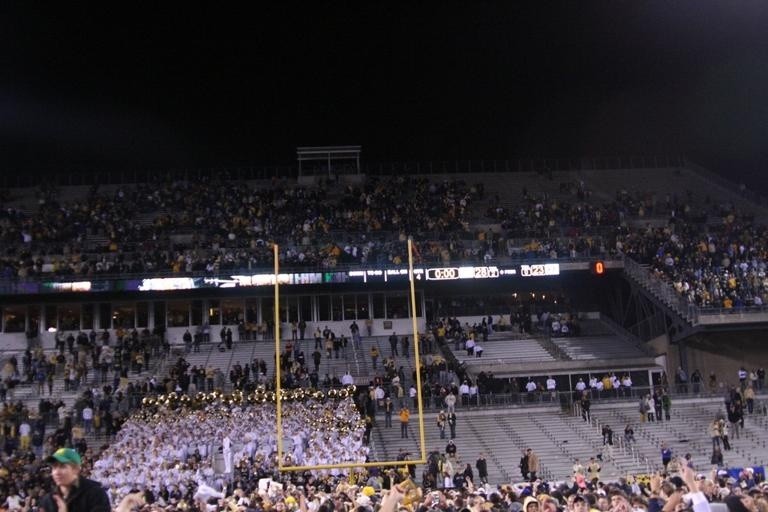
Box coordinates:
[0,172,766,489]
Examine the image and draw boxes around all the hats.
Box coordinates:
[43,447,80,466]
[718,470,729,477]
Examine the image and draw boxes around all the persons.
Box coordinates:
[0,162,768,512]
